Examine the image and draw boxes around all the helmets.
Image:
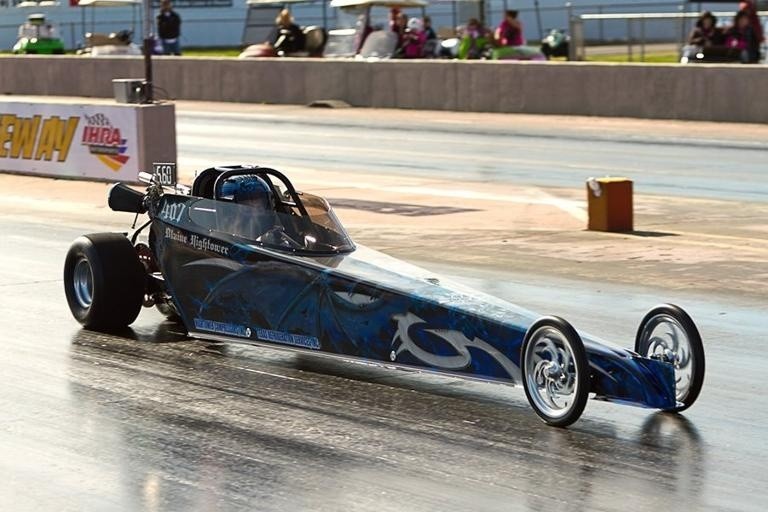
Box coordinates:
[408,17,424,31]
[217,174,274,216]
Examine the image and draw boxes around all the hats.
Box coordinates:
[696,12,716,27]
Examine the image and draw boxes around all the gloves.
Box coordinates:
[261,226,290,247]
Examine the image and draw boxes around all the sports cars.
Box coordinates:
[63,164,705,428]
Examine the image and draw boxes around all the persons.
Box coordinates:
[267,9,306,56]
[386,7,524,59]
[689,2,764,62]
[157,0,182,55]
[214,175,274,239]
